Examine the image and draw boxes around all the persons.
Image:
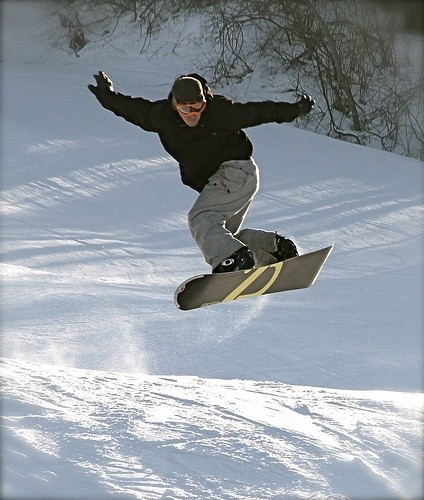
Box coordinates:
[88,71,316,274]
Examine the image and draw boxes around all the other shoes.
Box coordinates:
[213,246,255,274]
[275,236,299,263]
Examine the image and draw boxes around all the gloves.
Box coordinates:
[298,94,316,118]
[87,71,114,105]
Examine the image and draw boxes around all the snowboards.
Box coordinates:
[174,245,334,312]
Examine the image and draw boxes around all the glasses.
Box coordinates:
[172,95,206,117]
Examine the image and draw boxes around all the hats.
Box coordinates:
[172,77,203,102]
